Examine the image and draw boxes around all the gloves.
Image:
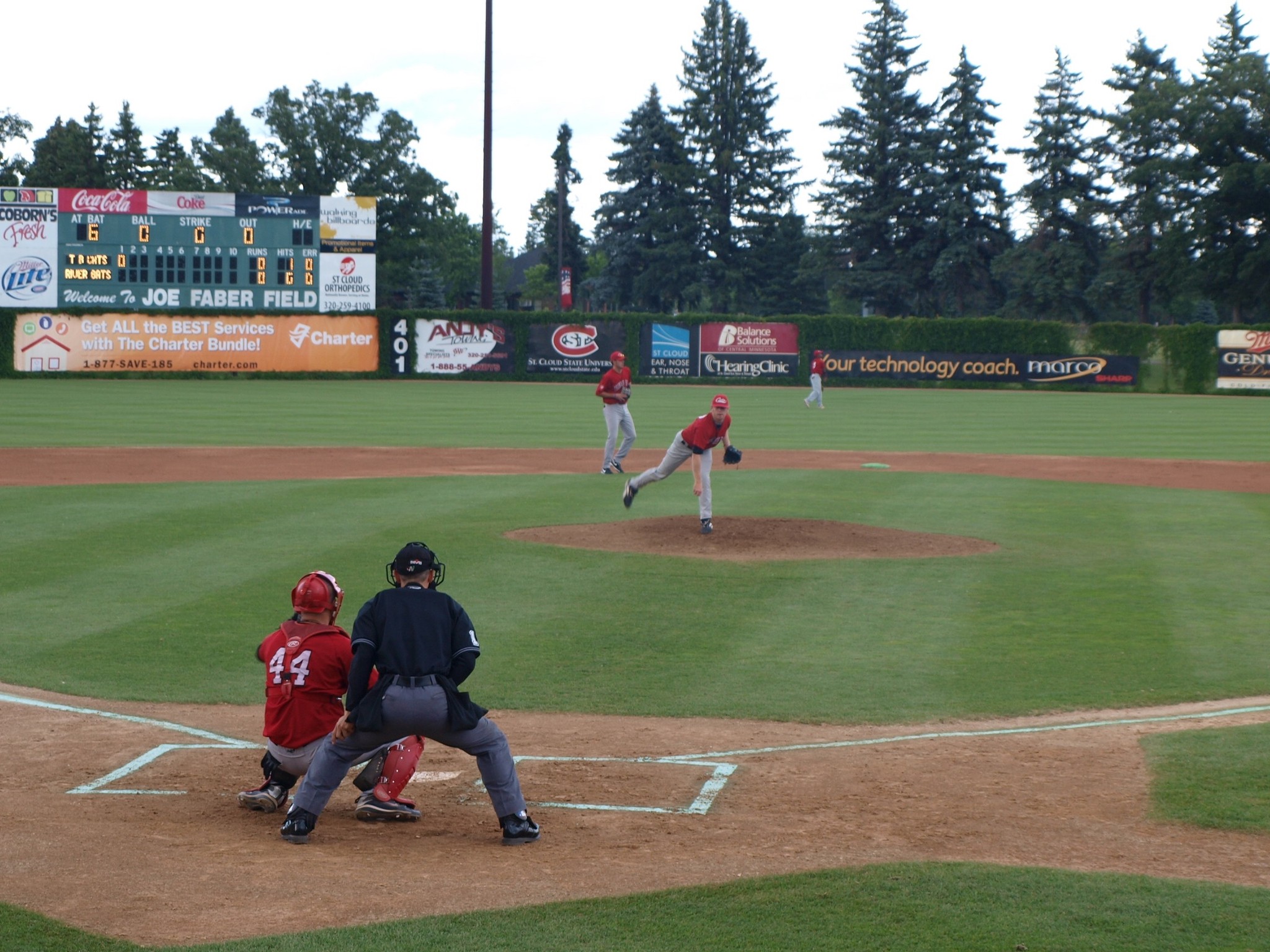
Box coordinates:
[261,749,280,780]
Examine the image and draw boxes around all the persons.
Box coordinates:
[280,541,541,845]
[622,393,742,534]
[237,571,425,821]
[596,351,636,475]
[804,350,827,409]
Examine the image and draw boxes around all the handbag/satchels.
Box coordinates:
[436,674,489,732]
[345,674,394,731]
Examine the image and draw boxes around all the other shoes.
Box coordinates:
[817,404,825,409]
[280,818,309,844]
[804,398,809,407]
[501,823,540,844]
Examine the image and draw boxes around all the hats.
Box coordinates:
[610,351,626,360]
[813,350,824,356]
[711,394,730,409]
[395,544,432,575]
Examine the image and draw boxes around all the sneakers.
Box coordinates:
[700,520,713,534]
[238,781,289,813]
[355,792,420,821]
[610,459,624,474]
[623,479,638,508]
[601,468,613,474]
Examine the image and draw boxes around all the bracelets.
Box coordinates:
[614,393,617,398]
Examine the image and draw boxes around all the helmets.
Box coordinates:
[292,571,344,626]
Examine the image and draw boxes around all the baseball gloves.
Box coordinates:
[724,446,743,469]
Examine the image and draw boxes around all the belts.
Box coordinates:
[681,441,693,450]
[389,675,438,688]
